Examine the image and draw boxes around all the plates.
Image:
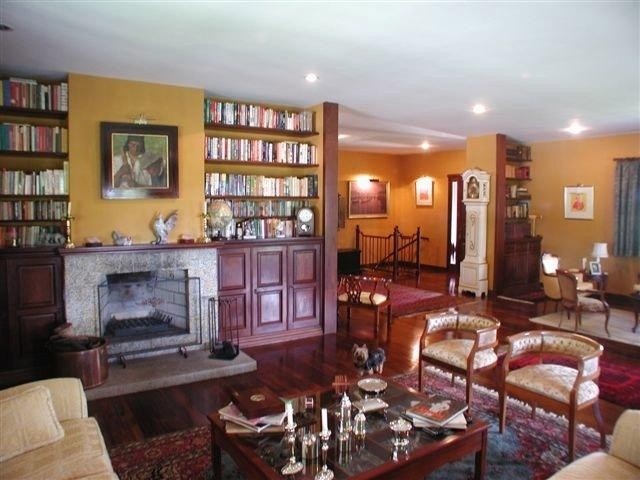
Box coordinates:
[357,377,387,392]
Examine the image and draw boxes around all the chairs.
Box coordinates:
[336,273,393,338]
[556,268,610,337]
[540,250,571,320]
[629,272,640,333]
[499,329,606,464]
[418,310,501,414]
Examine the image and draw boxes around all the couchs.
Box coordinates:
[545,408,640,480]
[0,377,120,480]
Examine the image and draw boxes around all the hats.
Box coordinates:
[140,151,162,171]
[124,136,144,152]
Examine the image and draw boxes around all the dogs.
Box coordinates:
[350,342,385,376]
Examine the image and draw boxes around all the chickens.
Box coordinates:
[149,209,178,245]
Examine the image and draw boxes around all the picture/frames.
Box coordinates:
[348,180,390,219]
[590,261,602,275]
[563,186,594,220]
[100,121,179,200]
[415,180,434,207]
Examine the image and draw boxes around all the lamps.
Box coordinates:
[591,242,609,262]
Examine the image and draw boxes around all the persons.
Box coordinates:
[114,136,169,190]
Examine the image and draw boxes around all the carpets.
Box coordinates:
[337,277,480,319]
[528,305,640,348]
[106,365,613,480]
[496,335,640,411]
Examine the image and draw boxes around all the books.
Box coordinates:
[0,199,69,221]
[0,122,67,154]
[0,226,65,245]
[505,205,530,216]
[217,388,288,433]
[203,98,316,133]
[0,77,69,110]
[504,143,531,159]
[230,219,294,238]
[204,136,316,164]
[226,199,311,216]
[401,394,468,431]
[503,165,530,180]
[506,184,528,197]
[205,172,318,198]
[1,161,69,194]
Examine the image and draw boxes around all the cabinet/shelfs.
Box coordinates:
[0,249,69,374]
[204,95,320,241]
[504,223,542,290]
[502,145,542,221]
[215,236,323,347]
[0,76,69,251]
